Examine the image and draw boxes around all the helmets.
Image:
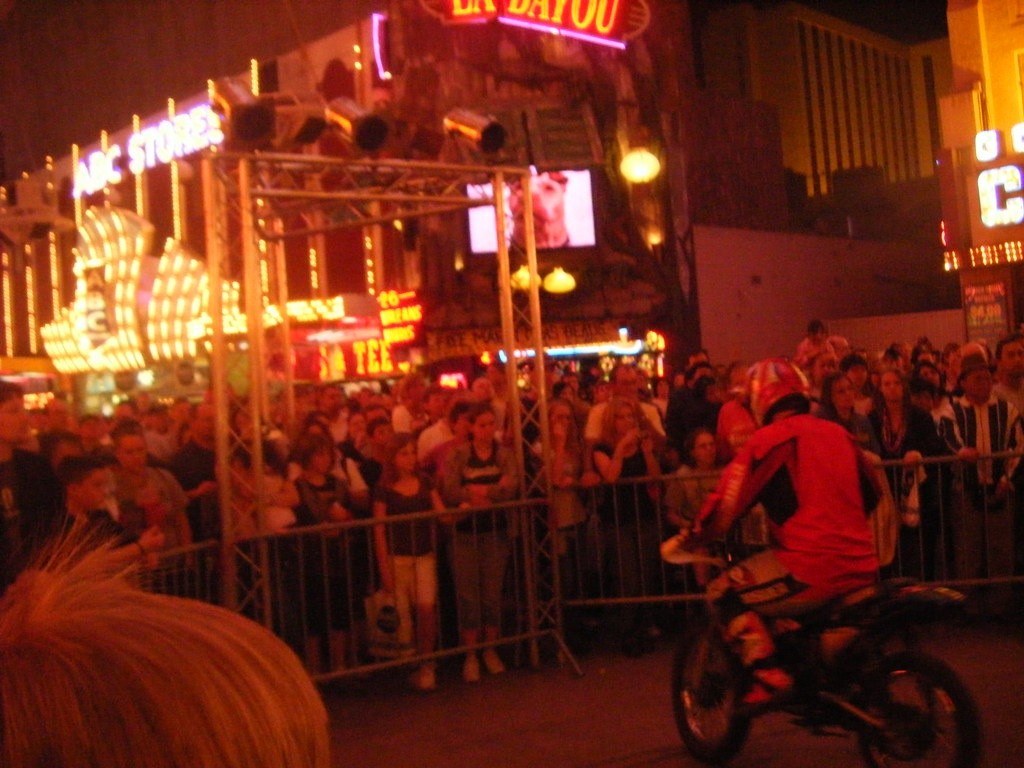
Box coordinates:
[746,357,810,426]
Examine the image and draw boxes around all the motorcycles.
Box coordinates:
[658,516,985,768]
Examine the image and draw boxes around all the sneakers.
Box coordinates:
[461,654,481,684]
[482,648,505,675]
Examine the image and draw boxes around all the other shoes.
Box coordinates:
[729,662,801,721]
[405,668,437,693]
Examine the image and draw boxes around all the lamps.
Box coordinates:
[325,97,388,155]
[207,74,272,149]
[443,109,507,161]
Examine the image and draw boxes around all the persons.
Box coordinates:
[0,315,1023,768]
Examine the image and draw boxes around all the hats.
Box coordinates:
[839,353,868,372]
[957,342,997,384]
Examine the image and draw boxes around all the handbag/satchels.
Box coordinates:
[363,589,416,659]
[899,462,921,526]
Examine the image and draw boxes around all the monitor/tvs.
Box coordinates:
[467,170,595,253]
